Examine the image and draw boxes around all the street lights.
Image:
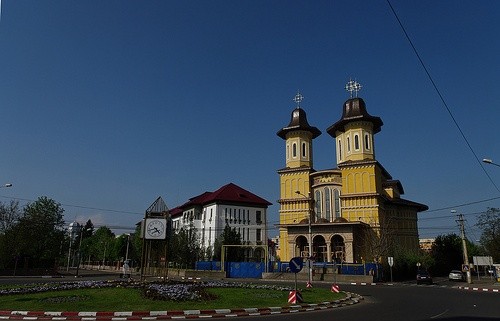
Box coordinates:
[295,191,313,281]
[76,227,91,276]
[451,210,472,284]
[123,234,130,278]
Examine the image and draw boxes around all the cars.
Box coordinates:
[416,272,432,285]
[449,270,466,282]
[471,267,494,276]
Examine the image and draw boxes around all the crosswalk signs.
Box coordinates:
[462,264,469,272]
